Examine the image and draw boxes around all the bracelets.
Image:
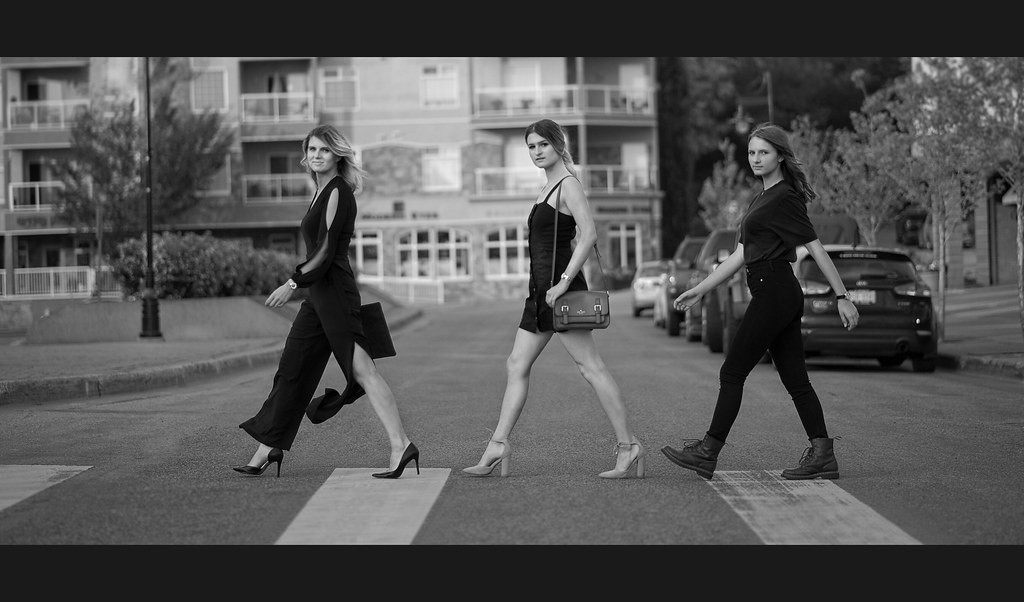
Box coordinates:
[560,273,574,281]
[288,280,298,292]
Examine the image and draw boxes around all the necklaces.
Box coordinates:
[542,168,566,193]
[761,176,783,196]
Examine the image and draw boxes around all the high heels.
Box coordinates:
[372,442,420,479]
[462,438,512,477]
[232,448,284,478]
[599,438,645,479]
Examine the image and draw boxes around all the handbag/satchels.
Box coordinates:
[551,290,610,331]
[362,302,396,360]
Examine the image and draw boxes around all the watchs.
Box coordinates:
[836,292,851,301]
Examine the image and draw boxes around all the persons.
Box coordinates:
[462,120,647,479]
[661,125,861,481]
[233,126,420,479]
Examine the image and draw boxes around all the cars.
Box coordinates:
[631,227,940,372]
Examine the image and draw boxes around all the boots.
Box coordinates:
[781,438,840,480]
[660,433,725,480]
